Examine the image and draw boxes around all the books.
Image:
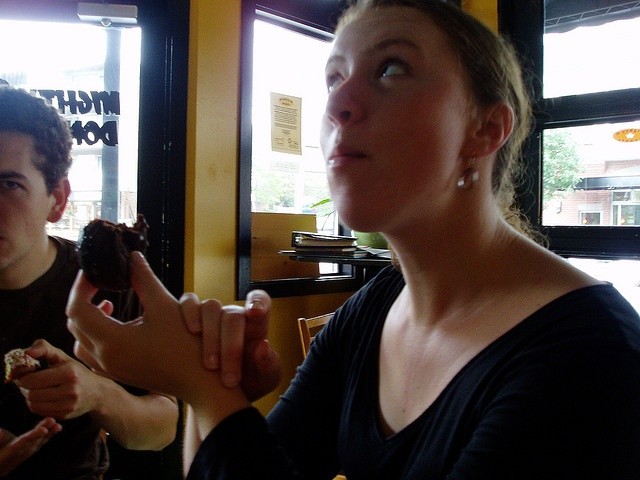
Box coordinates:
[276,231,369,259]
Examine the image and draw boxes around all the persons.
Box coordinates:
[0,86,179,480]
[64,0,640,479]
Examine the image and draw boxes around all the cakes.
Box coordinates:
[78,216,149,291]
[3,348,41,383]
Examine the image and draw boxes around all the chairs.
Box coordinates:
[296,309,334,357]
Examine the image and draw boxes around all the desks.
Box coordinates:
[276,246,398,277]
